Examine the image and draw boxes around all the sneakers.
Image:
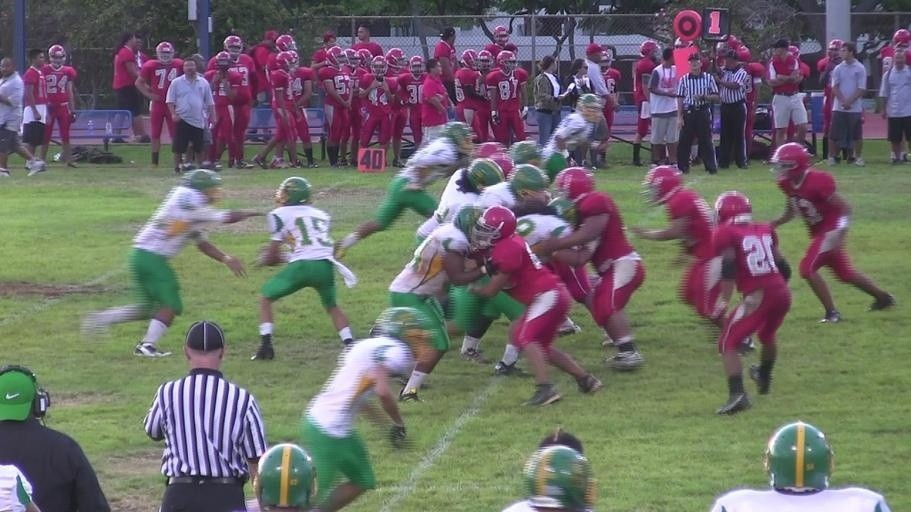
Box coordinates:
[524,385,558,405]
[870,295,893,312]
[749,363,770,394]
[578,372,601,396]
[740,338,755,355]
[464,349,487,364]
[718,391,751,413]
[3,159,50,176]
[133,341,172,358]
[495,362,522,377]
[172,156,404,173]
[250,346,274,361]
[818,310,840,324]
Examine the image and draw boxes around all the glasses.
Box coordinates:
[584,66,588,70]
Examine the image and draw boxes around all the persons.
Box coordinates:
[708,421,891,511]
[141,319,271,512]
[298,304,438,512]
[22,48,48,173]
[0,56,47,177]
[113,26,911,175]
[520,444,599,512]
[39,44,79,168]
[90,93,896,417]
[501,428,584,512]
[245,437,322,511]
[0,365,109,511]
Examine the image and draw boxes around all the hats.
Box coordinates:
[0,371,34,420]
[185,321,224,351]
[581,43,608,54]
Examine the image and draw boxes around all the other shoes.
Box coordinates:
[558,323,581,335]
[603,352,642,368]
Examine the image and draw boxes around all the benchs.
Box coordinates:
[336,106,462,159]
[524,105,653,157]
[712,102,818,155]
[48,109,133,151]
[247,108,329,161]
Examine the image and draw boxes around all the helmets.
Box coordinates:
[524,444,595,510]
[648,166,682,206]
[773,142,806,181]
[715,192,754,222]
[216,51,230,70]
[280,178,311,206]
[463,49,477,68]
[497,50,516,72]
[641,30,907,67]
[454,142,595,245]
[275,34,299,70]
[579,93,601,123]
[477,50,493,70]
[326,47,422,73]
[386,307,431,360]
[443,121,470,150]
[157,42,173,62]
[254,443,318,508]
[493,28,509,43]
[188,168,219,191]
[224,35,242,55]
[49,45,66,64]
[765,422,832,491]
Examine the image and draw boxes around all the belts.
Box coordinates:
[688,105,710,111]
[777,92,798,96]
[168,476,236,486]
